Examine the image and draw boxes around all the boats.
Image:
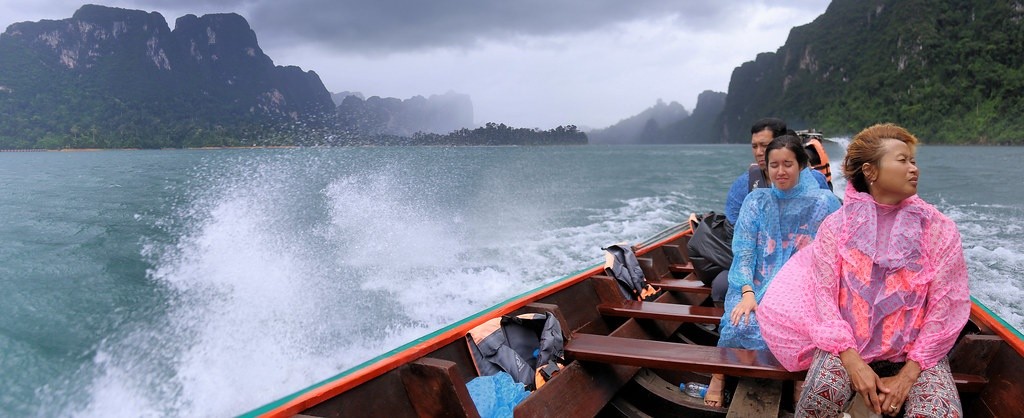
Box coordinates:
[234,128,1024,418]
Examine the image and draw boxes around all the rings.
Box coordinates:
[889,404,897,410]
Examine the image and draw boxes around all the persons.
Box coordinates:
[702,119,842,411]
[794,122,972,418]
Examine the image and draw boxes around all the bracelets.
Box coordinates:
[740,290,755,297]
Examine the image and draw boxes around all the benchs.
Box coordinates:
[563,261,988,392]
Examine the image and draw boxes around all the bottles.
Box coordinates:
[680,382,730,402]
